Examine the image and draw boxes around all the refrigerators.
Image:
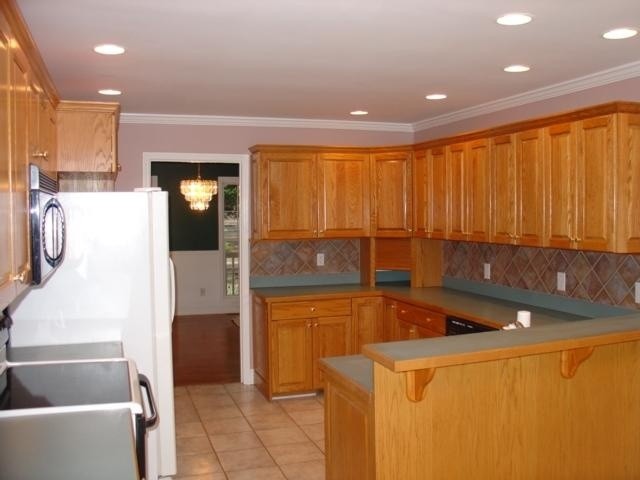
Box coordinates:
[7,187,179,480]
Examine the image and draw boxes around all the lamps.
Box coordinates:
[180,164,218,211]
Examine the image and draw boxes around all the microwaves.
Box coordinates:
[29,164,68,287]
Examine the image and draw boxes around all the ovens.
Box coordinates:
[0,374,158,480]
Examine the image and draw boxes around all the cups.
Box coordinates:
[516,310,531,328]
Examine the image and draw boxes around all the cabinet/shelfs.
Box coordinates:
[351,292,381,355]
[31,37,58,184]
[492,118,543,249]
[0,0,32,309]
[56,108,118,173]
[253,293,355,402]
[381,298,446,339]
[372,144,412,239]
[542,99,640,255]
[448,129,492,244]
[412,138,448,241]
[250,145,372,241]
